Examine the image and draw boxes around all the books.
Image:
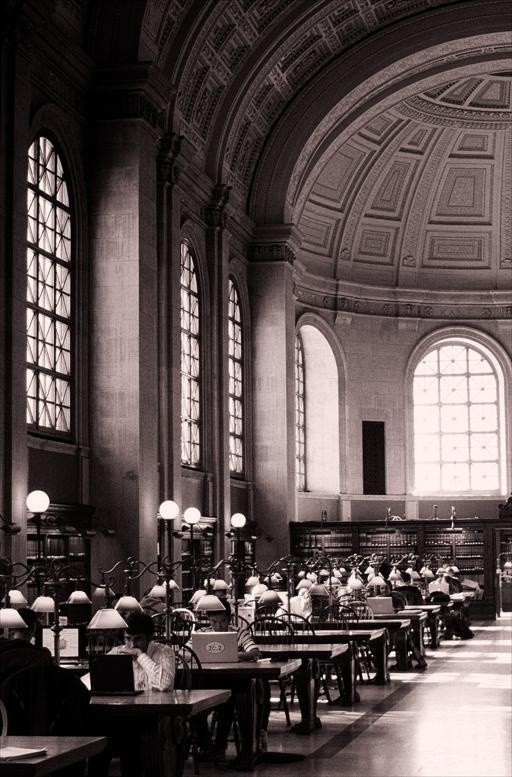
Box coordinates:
[292,523,485,602]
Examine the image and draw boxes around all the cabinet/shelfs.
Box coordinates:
[420,520,495,620]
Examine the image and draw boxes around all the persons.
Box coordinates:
[110,561,465,690]
[0,607,91,737]
[78,613,175,776]
[178,599,270,769]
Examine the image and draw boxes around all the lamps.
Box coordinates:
[496,552,512,574]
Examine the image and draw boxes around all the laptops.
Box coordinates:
[367,596,396,614]
[429,583,450,595]
[89,655,144,696]
[192,632,241,663]
[42,628,80,664]
